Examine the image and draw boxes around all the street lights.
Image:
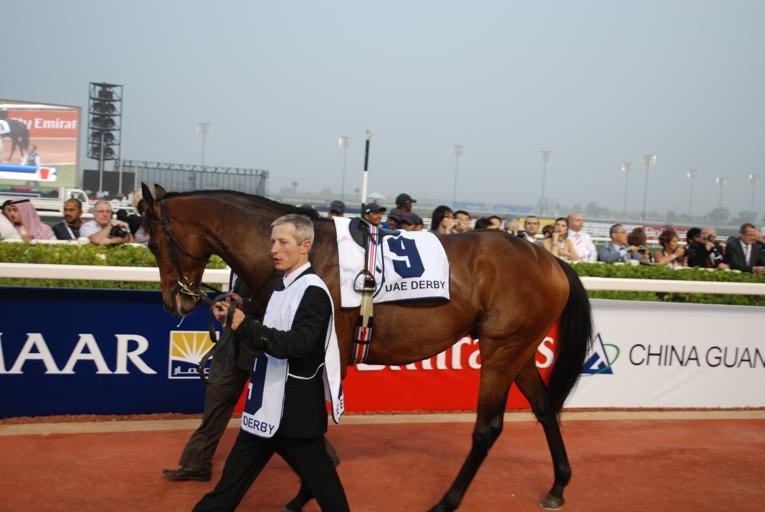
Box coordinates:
[537,149,553,215]
[86,82,123,198]
[450,142,464,210]
[337,135,353,203]
[621,153,760,225]
[193,121,213,190]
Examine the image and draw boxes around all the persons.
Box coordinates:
[190,215,351,511]
[162,276,341,480]
[0,193,150,247]
[21,145,40,186]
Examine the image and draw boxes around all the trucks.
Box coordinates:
[20,186,91,215]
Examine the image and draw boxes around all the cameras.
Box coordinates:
[682,247,691,257]
[111,226,127,237]
[451,222,459,228]
[712,246,723,252]
[638,248,645,253]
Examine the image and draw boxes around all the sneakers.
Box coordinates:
[162,464,212,483]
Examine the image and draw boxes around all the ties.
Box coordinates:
[745,246,751,266]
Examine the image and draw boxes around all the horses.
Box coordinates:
[0,119,30,161]
[134,179,594,512]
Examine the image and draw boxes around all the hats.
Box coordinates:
[329,200,346,215]
[361,193,421,225]
